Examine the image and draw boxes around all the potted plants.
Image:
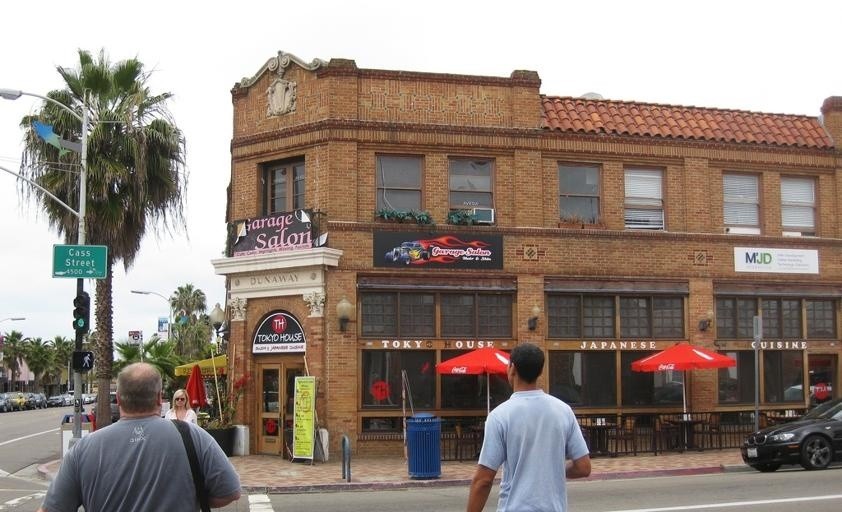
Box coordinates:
[583,216,607,230]
[558,213,583,229]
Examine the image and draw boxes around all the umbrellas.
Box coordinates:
[631,342,737,451]
[186,364,206,413]
[435,346,511,416]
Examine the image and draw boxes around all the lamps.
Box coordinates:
[336,295,352,333]
[209,303,230,337]
[699,307,713,331]
[216,335,227,354]
[528,302,539,330]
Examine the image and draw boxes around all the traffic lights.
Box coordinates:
[71,350,95,370]
[73,292,90,334]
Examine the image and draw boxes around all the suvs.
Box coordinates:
[793,368,832,387]
[91,391,120,422]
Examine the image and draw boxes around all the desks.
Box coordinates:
[580,422,619,455]
[770,416,802,424]
[671,420,702,451]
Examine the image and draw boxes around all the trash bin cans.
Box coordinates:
[61,414,93,467]
[405,413,441,481]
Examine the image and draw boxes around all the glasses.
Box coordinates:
[176,398,184,401]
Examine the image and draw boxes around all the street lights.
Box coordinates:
[0,88,89,440]
[131,290,172,344]
[1,316,27,323]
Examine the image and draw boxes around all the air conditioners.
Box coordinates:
[473,208,494,225]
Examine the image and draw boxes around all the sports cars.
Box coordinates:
[383,240,435,265]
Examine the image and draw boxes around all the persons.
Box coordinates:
[37,362,242,512]
[165,389,197,424]
[466,343,591,512]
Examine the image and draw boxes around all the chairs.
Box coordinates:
[455,422,478,463]
[702,412,722,451]
[655,415,682,456]
[607,414,636,457]
[758,412,768,429]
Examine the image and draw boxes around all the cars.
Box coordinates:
[1,391,97,412]
[740,398,842,472]
[394,369,509,408]
[767,384,832,402]
[550,384,580,404]
[653,381,685,404]
[719,377,737,400]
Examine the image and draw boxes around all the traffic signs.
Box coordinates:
[51,243,108,280]
